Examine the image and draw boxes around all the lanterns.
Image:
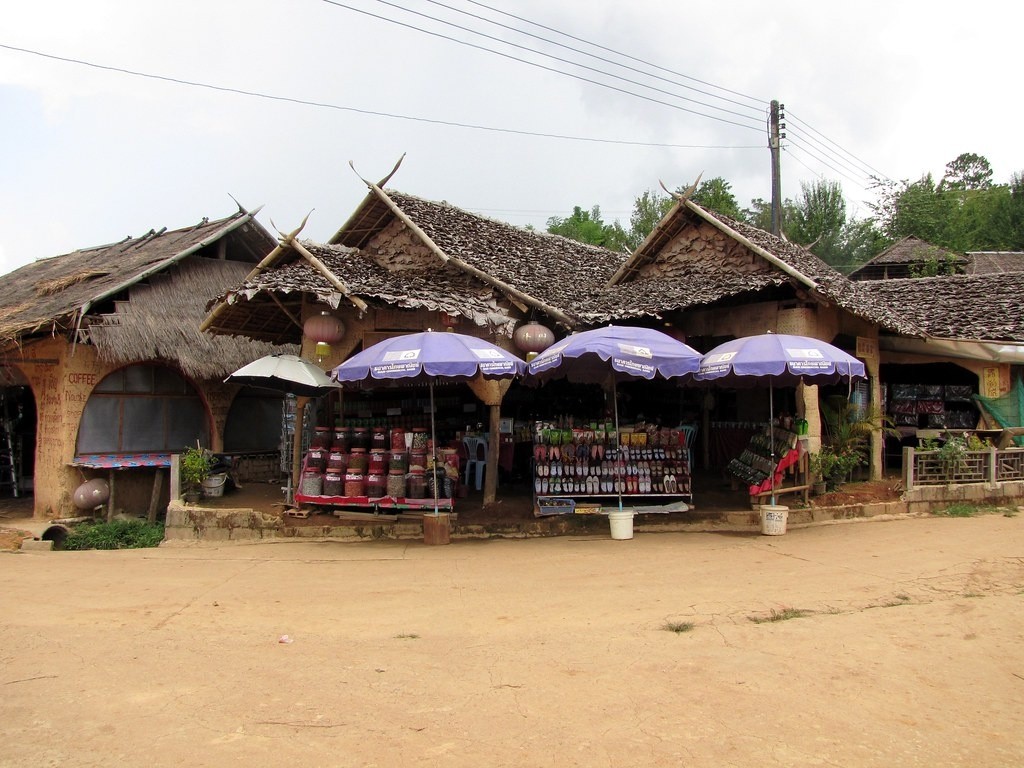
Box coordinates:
[304,311,345,357]
[514,321,555,363]
[657,322,685,343]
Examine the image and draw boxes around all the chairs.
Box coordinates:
[461,436,499,490]
[675,426,697,460]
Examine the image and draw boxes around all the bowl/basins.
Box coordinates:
[436,447,457,454]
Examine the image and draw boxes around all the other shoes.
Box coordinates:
[534,445,689,493]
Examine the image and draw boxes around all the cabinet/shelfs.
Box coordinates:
[295,448,458,513]
[329,384,489,448]
[532,438,693,508]
[728,417,810,506]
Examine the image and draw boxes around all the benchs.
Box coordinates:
[915,427,1024,485]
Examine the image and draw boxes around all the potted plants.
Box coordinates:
[182,446,218,505]
[809,397,901,494]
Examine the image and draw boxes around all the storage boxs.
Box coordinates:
[537,498,576,513]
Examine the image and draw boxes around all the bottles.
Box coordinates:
[387,470,406,497]
[311,427,330,452]
[371,428,387,449]
[407,471,427,498]
[331,427,350,452]
[389,449,406,472]
[331,379,484,441]
[391,428,405,450]
[352,428,370,452]
[369,449,385,474]
[373,503,379,514]
[344,468,364,496]
[349,448,368,475]
[323,468,343,496]
[366,469,385,497]
[328,448,344,473]
[409,449,426,471]
[302,468,322,495]
[308,446,324,473]
[411,427,428,449]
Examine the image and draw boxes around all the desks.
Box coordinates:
[456,438,532,494]
[941,428,1003,482]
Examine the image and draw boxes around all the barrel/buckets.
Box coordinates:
[759,504,788,536]
[202,472,226,497]
[609,510,634,540]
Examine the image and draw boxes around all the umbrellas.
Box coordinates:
[223,354,343,398]
[329,328,528,513]
[529,323,704,512]
[694,331,868,506]
